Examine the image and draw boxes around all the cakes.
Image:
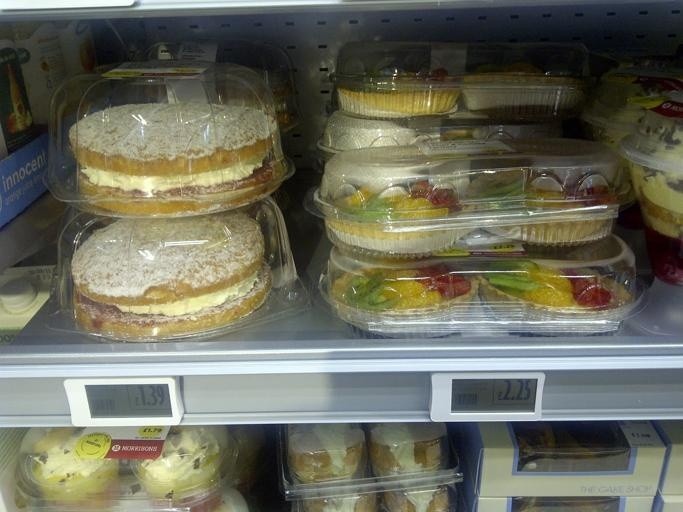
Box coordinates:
[321,62,683,341]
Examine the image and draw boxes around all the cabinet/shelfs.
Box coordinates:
[0,1,683,511]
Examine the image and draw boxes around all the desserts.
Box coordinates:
[70,77,297,340]
[29,422,266,512]
[285,424,450,511]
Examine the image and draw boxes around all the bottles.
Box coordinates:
[0,40,36,152]
